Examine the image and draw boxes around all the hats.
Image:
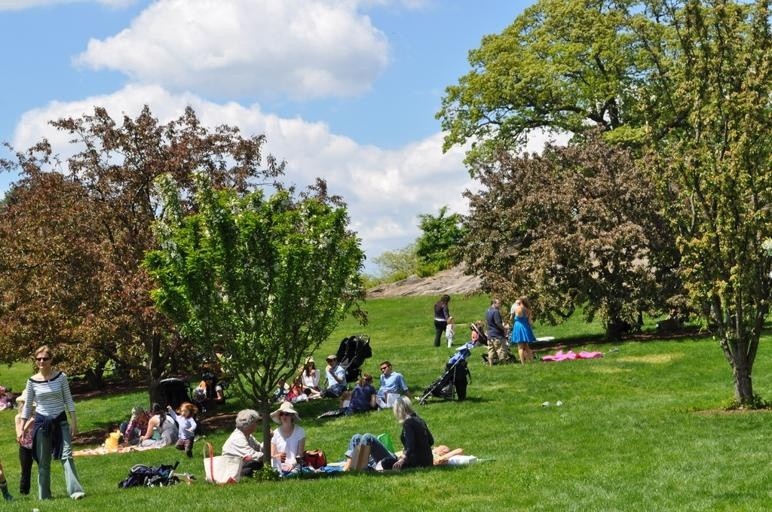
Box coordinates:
[269,402,299,424]
[326,355,336,360]
[304,356,314,365]
[15,389,27,402]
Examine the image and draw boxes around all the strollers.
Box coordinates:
[334,335,372,385]
[159,378,202,415]
[420,343,471,404]
[470,320,492,348]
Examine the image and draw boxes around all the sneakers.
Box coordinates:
[70,492,85,499]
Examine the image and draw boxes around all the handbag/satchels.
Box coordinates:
[304,450,327,469]
[202,442,252,485]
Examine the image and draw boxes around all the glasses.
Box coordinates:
[381,364,391,371]
[279,412,289,416]
[327,360,332,363]
[36,358,49,361]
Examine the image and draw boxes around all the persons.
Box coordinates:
[0,462,14,502]
[14,387,40,495]
[344,373,378,415]
[17,345,85,501]
[472,295,536,367]
[275,355,352,411]
[267,401,306,479]
[445,316,455,348]
[343,433,464,472]
[393,395,433,470]
[376,361,412,405]
[120,373,223,457]
[433,294,450,346]
[221,408,285,478]
